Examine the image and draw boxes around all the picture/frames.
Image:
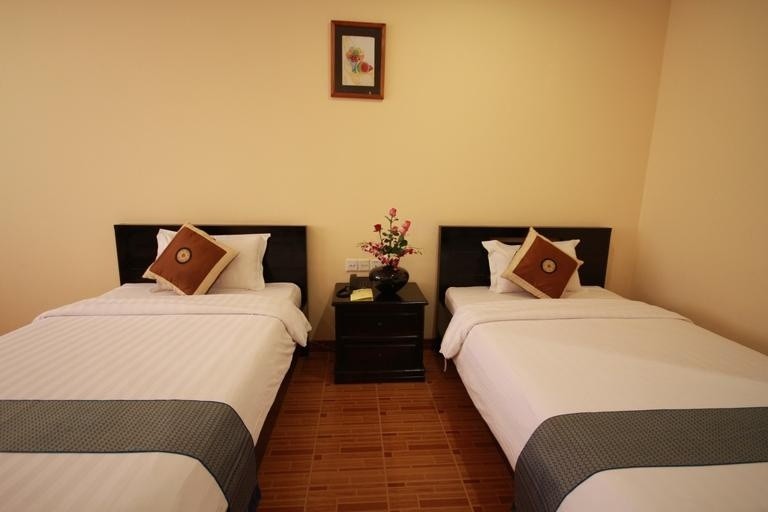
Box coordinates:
[331,20,386,99]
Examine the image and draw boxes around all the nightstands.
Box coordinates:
[332,283,429,384]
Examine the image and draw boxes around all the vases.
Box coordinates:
[368,265,409,294]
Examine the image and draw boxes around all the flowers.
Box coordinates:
[353,208,425,271]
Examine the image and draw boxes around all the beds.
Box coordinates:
[435,225,768,512]
[0,226,306,512]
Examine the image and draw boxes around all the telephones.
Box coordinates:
[350,274,373,290]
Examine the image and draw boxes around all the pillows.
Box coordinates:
[141,221,271,295]
[482,225,584,299]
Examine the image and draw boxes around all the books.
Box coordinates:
[350,288,373,303]
